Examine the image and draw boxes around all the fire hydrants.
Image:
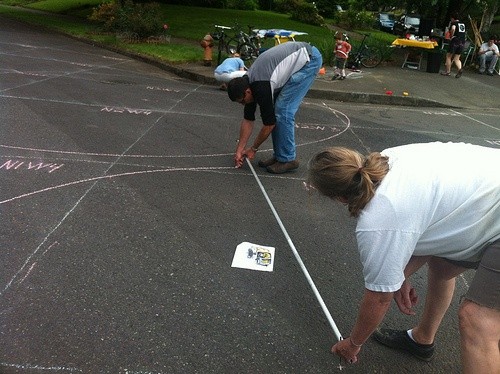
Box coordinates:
[199,32,214,67]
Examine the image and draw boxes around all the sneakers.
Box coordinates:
[374,327,435,362]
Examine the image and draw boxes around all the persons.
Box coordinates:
[478,36,500,76]
[214,53,247,89]
[440,11,466,78]
[227,42,323,174]
[331,31,352,80]
[306,140,500,374]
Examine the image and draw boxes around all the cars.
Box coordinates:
[374,12,434,39]
[322,4,348,19]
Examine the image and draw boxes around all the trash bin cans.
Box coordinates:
[425,49,444,73]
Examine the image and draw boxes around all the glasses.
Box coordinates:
[242,95,246,106]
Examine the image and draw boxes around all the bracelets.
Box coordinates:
[350,337,363,347]
[251,147,258,151]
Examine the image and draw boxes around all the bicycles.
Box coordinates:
[329,32,384,69]
[209,23,261,72]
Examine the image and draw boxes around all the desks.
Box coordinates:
[392,39,439,71]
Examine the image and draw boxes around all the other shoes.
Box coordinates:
[455,69,463,79]
[258,157,300,174]
[440,71,451,76]
[340,76,345,80]
[489,72,494,76]
[332,73,339,81]
[478,71,484,74]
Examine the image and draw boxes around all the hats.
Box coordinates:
[334,32,345,41]
[233,53,239,58]
